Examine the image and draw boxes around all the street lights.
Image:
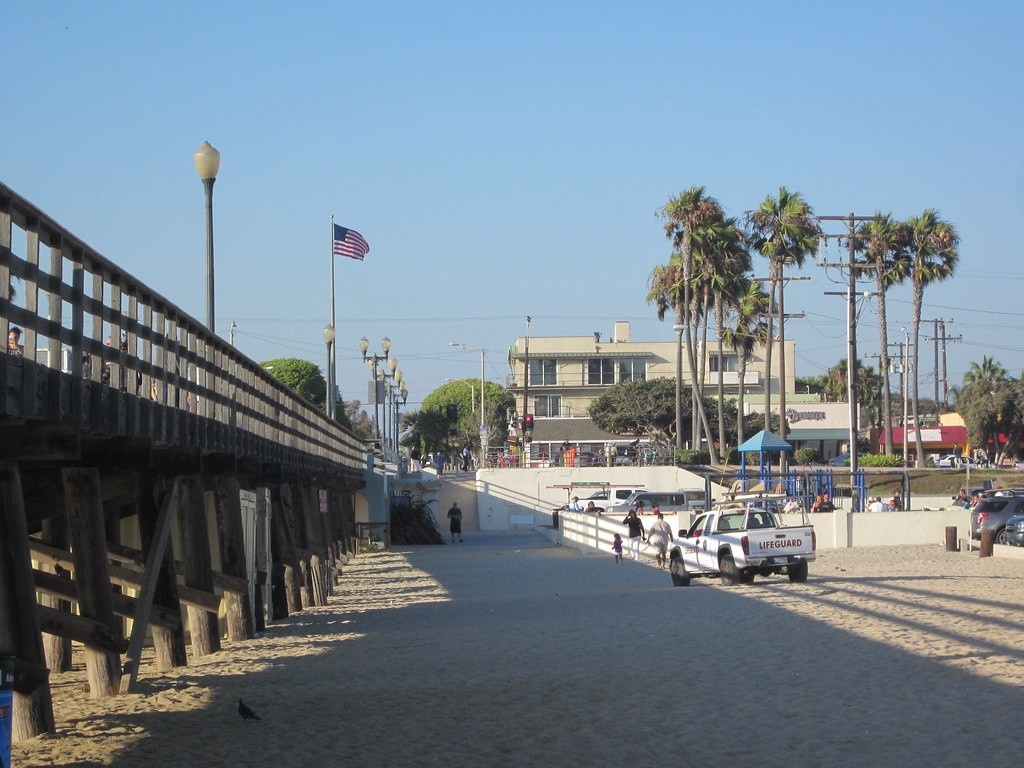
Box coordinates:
[195,140,221,332]
[384,380,405,452]
[380,369,404,449]
[673,325,787,505]
[358,335,392,446]
[450,342,485,468]
[392,389,408,453]
[846,289,874,499]
[369,357,398,441]
[445,378,474,413]
[323,323,336,417]
[901,326,909,468]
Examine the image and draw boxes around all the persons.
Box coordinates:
[433,452,444,478]
[569,496,579,511]
[9,327,25,357]
[586,501,594,512]
[629,438,640,462]
[462,444,470,471]
[599,442,618,466]
[636,501,644,515]
[973,445,989,468]
[410,445,420,473]
[653,505,660,515]
[102,337,142,394]
[867,490,900,512]
[784,498,800,513]
[560,439,570,452]
[623,510,645,561]
[811,493,835,513]
[958,489,972,509]
[611,534,624,565]
[952,443,962,468]
[647,513,673,569]
[447,502,464,543]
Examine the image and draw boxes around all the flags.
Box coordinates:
[333,223,370,261]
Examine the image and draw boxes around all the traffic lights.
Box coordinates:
[512,417,519,428]
[524,414,534,430]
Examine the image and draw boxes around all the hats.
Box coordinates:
[869,497,874,501]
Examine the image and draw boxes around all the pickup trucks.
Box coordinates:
[576,487,651,512]
[668,501,817,587]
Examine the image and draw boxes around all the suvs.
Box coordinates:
[952,479,1024,547]
[678,487,718,514]
[604,491,689,515]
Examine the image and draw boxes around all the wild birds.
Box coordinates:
[238,696,260,723]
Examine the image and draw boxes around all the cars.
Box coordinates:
[829,451,868,467]
[906,453,1024,471]
[571,444,669,467]
